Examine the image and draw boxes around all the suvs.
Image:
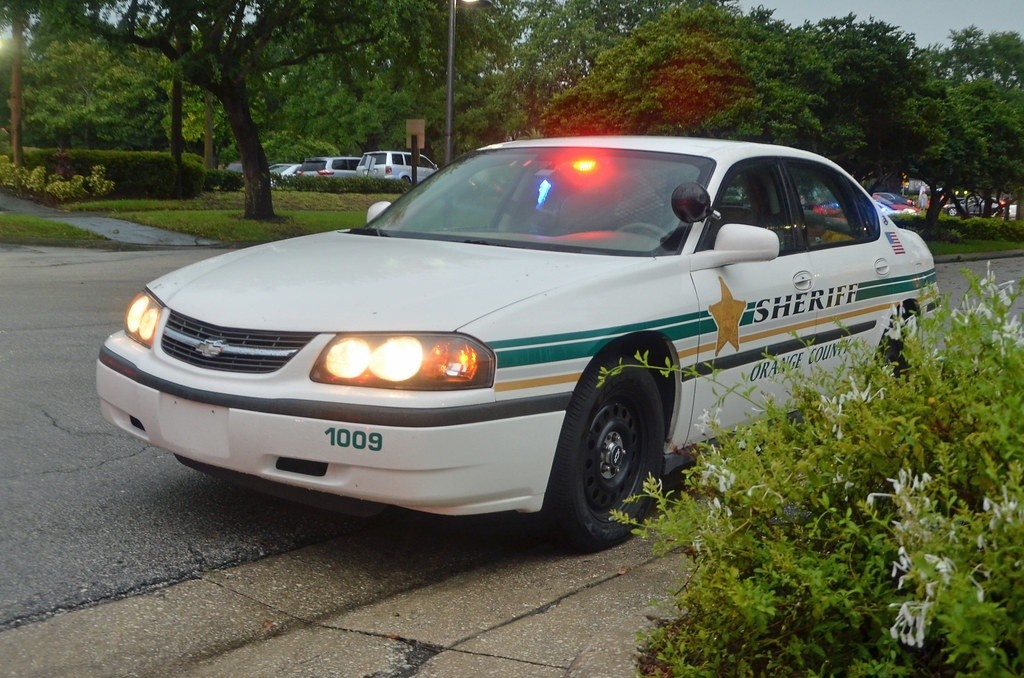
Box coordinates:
[299,156,360,180]
[355,150,440,186]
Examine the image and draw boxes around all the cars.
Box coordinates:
[226,160,244,188]
[805,177,1006,221]
[267,163,302,187]
[96,134,936,555]
